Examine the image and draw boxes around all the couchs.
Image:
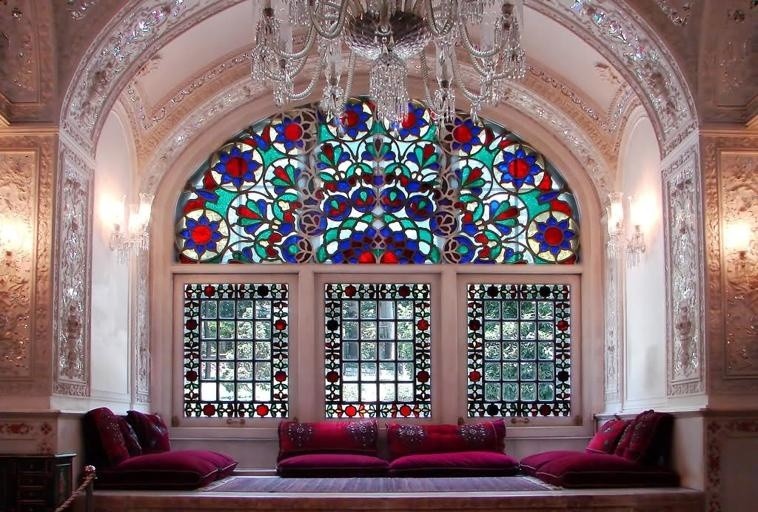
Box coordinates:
[97,447,241,490]
[275,452,392,480]
[387,449,521,478]
[519,442,682,491]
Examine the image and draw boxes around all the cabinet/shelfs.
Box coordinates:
[1,452,77,511]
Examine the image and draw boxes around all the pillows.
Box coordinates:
[384,417,509,456]
[582,407,675,466]
[277,417,380,460]
[81,403,172,468]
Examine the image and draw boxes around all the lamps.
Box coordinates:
[245,0,528,133]
[106,188,157,261]
[594,189,650,269]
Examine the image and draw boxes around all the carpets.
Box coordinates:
[208,476,550,493]
